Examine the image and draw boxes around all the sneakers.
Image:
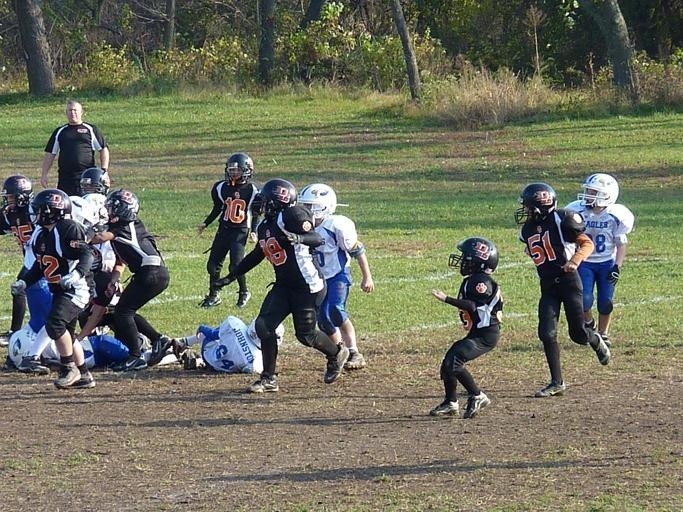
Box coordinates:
[589,330,611,365]
[53,361,82,389]
[245,372,280,394]
[343,348,367,370]
[324,341,351,384]
[17,354,51,376]
[106,354,148,374]
[171,337,189,365]
[236,288,252,308]
[67,371,96,389]
[0,328,15,348]
[534,379,566,398]
[196,290,223,309]
[181,348,201,371]
[463,389,492,420]
[597,331,612,347]
[429,399,460,418]
[147,334,173,366]
[3,356,16,372]
[583,318,597,332]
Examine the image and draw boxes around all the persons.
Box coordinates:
[197,154,259,308]
[212,178,349,392]
[430,237,503,420]
[564,174,634,347]
[94,189,173,372]
[76,168,115,341]
[84,284,152,354]
[298,184,374,369]
[8,194,92,373]
[11,188,101,389]
[1,175,38,347]
[172,315,285,373]
[41,101,110,193]
[513,183,610,396]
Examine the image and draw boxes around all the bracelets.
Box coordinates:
[103,168,108,172]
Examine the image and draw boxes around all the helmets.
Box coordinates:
[26,188,72,226]
[0,176,34,210]
[224,153,254,184]
[8,328,41,371]
[447,237,500,277]
[253,178,298,220]
[78,167,111,197]
[514,182,558,225]
[103,188,140,226]
[577,172,620,209]
[296,183,338,219]
[247,314,285,350]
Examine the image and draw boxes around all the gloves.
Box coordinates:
[212,277,231,292]
[59,269,81,290]
[281,227,303,247]
[606,264,620,287]
[10,280,27,297]
